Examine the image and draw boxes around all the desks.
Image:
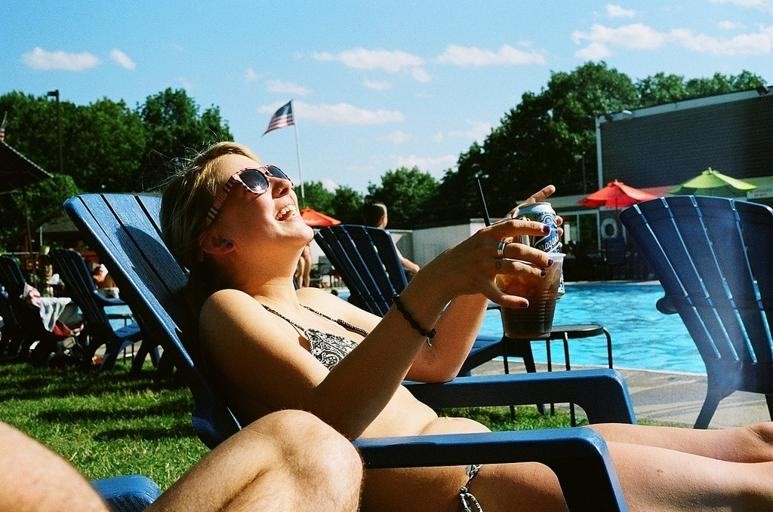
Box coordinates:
[544,323,613,427]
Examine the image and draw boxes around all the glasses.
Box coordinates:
[206,164,294,228]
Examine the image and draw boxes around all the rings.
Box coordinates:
[496,240,510,260]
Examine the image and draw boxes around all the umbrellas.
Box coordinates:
[576,177,658,233]
[667,166,758,198]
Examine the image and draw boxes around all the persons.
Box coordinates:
[2,242,134,363]
[161,142,772,511]
[0,409,363,512]
[294,204,421,307]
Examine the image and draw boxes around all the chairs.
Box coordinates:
[1,256,88,364]
[48,249,160,377]
[620,194,771,428]
[64,191,638,512]
[313,224,517,421]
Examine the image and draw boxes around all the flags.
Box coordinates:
[263,99,294,136]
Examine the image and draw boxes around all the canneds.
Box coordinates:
[517,202,565,295]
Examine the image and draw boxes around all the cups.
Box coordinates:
[495,252,564,340]
